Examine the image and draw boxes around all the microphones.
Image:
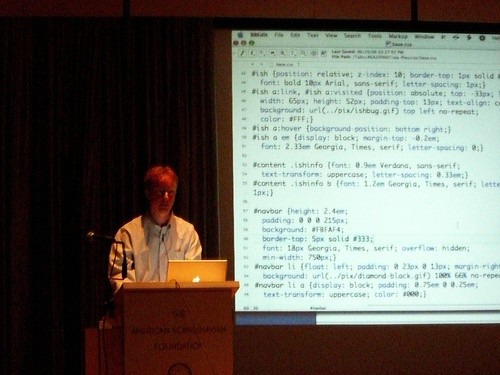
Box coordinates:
[86,232,116,242]
[161,237,164,242]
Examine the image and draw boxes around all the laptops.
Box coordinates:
[166,259,227,282]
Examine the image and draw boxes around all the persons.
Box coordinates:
[108,166,202,294]
